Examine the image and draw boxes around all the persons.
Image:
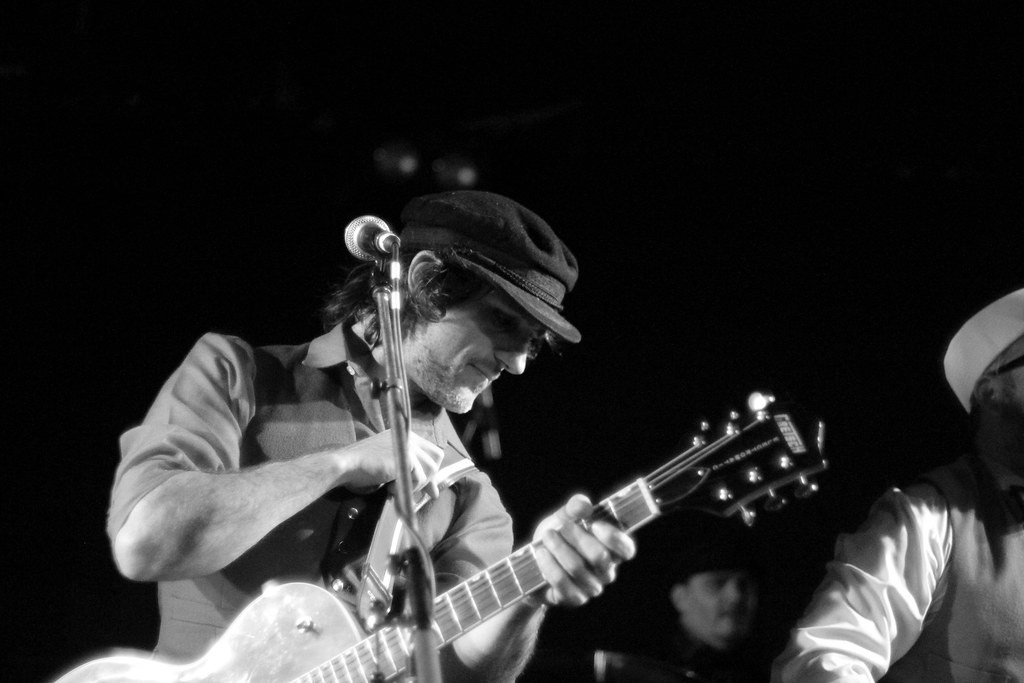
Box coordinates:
[106,190,639,683]
[770,287,1024,683]
[645,546,769,682]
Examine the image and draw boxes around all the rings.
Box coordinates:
[596,552,611,574]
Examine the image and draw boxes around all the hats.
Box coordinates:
[943,288,1024,414]
[403,189,582,343]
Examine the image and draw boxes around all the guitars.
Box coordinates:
[51,391,826,683]
